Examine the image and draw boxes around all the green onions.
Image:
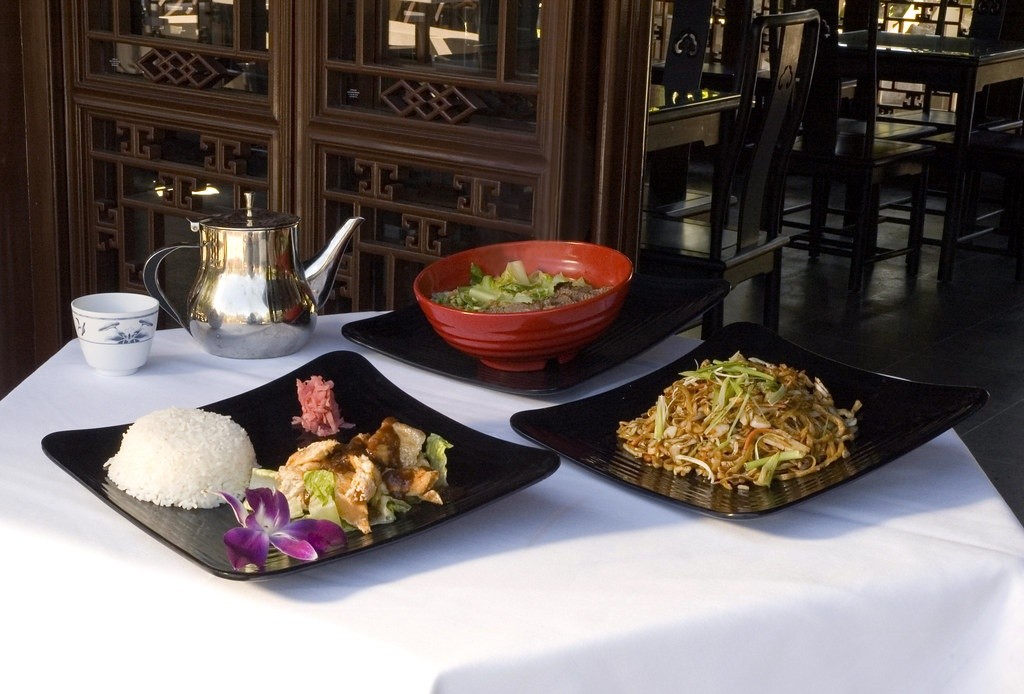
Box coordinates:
[655,359,808,488]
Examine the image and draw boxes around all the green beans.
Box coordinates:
[429,260,588,311]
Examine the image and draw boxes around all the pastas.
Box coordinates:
[617,352,861,488]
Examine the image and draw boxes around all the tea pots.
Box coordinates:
[142,190,365,360]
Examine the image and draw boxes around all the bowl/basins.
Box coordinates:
[412,240,635,371]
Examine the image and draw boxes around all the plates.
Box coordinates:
[511,321,991,519]
[341,268,729,397]
[41,350,562,582]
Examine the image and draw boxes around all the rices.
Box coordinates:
[103,407,261,510]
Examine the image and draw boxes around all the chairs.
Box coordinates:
[769,1,937,227]
[874,1,1024,216]
[936,130,1023,284]
[639,8,824,344]
[647,1,754,224]
[782,0,930,295]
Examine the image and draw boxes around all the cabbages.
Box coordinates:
[426,432,455,487]
[302,470,335,506]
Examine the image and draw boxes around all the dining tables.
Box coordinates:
[1,312,1024,691]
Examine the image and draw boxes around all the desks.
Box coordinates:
[648,83,741,152]
[799,28,1024,282]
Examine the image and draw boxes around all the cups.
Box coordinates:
[70,292,160,378]
